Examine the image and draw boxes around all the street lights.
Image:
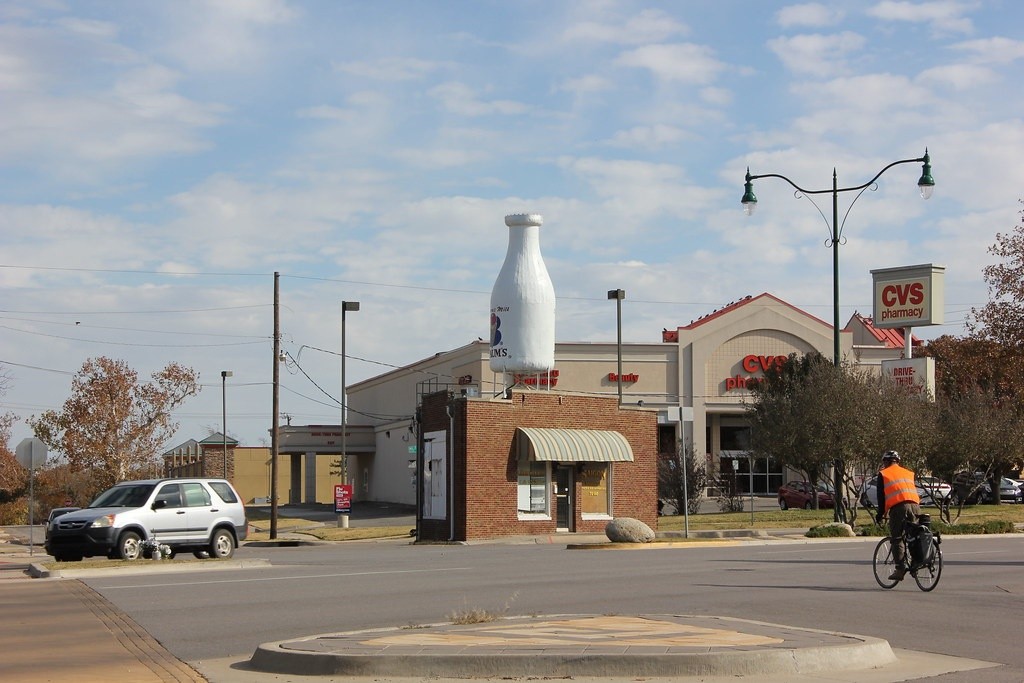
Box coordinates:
[337,301,362,528]
[220,370,234,479]
[607,288,627,404]
[739,147,937,515]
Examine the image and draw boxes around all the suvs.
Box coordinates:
[45,476,249,563]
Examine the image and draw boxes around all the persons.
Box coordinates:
[875,451,920,582]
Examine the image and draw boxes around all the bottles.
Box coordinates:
[489,214,556,370]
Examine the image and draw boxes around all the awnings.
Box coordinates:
[517,427,634,463]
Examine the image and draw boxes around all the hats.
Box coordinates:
[883,450,900,462]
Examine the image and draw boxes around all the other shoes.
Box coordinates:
[888,571,904,581]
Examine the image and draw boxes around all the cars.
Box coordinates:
[857,474,1024,509]
[44,507,83,539]
[777,480,848,511]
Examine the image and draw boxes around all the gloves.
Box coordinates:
[876,514,882,523]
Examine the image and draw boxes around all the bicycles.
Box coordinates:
[871,515,944,594]
